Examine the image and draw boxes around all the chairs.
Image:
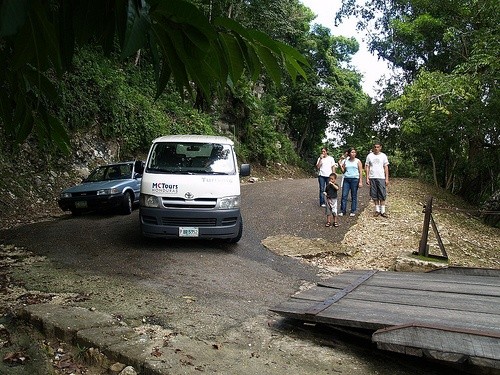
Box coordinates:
[108,169,120,181]
[204,148,225,171]
[157,146,183,174]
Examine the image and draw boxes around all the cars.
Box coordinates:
[60,160,145,217]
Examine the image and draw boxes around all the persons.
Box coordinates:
[366,143,390,218]
[316,147,335,207]
[338,148,352,202]
[323,173,340,227]
[338,147,363,217]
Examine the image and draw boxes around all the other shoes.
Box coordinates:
[321,204,326,207]
[325,222,331,227]
[333,222,339,227]
[349,213,355,216]
[374,211,380,217]
[338,213,344,216]
[381,213,387,217]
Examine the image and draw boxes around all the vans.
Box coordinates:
[140,135,249,243]
